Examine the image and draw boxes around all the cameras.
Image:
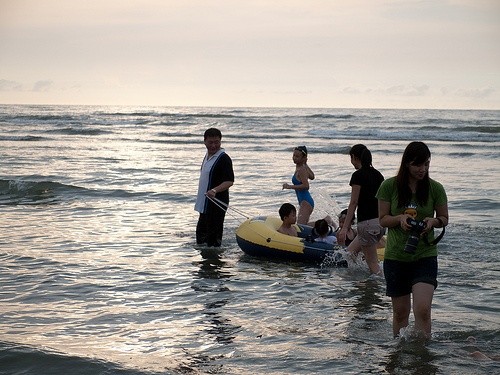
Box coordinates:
[403,217,427,255]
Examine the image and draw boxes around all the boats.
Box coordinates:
[235,216,389,266]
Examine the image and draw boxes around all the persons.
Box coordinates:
[279,203,298,237]
[468,350,492,362]
[312,219,339,245]
[339,143,385,276]
[336,209,387,252]
[282,145,337,229]
[375,142,449,337]
[193,127,235,248]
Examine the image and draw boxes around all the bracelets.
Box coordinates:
[211,189,216,194]
[292,185,296,189]
